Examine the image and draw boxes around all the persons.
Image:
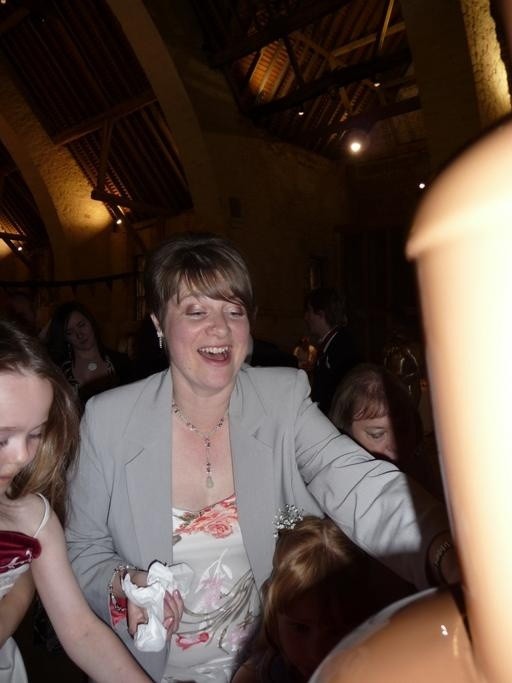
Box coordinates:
[63,237,464,683]
[1,302,157,682]
[231,289,464,683]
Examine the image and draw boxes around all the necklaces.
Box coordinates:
[172,401,229,489]
